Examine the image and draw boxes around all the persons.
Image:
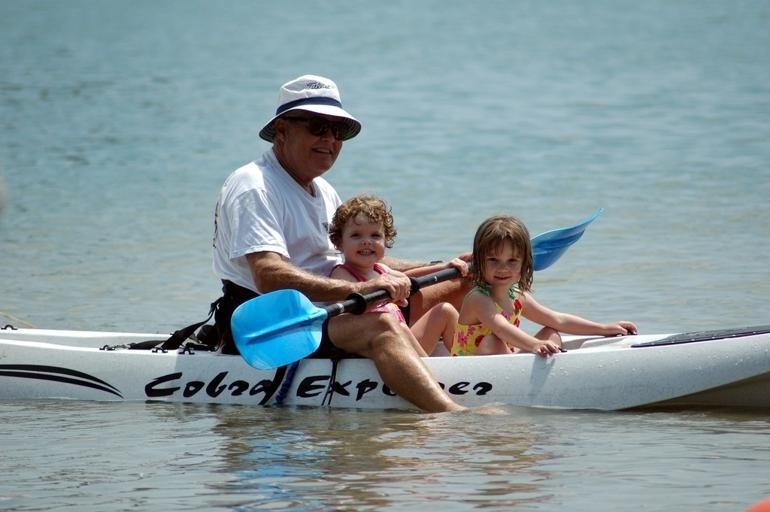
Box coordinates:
[325,194,459,357]
[211,74,506,418]
[447,214,637,355]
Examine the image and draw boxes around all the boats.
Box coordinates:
[0,323,769,410]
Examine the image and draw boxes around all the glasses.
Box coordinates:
[286,117,349,140]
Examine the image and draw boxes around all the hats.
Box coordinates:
[259,74,361,142]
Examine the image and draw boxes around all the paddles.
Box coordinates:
[230,209,603,370]
